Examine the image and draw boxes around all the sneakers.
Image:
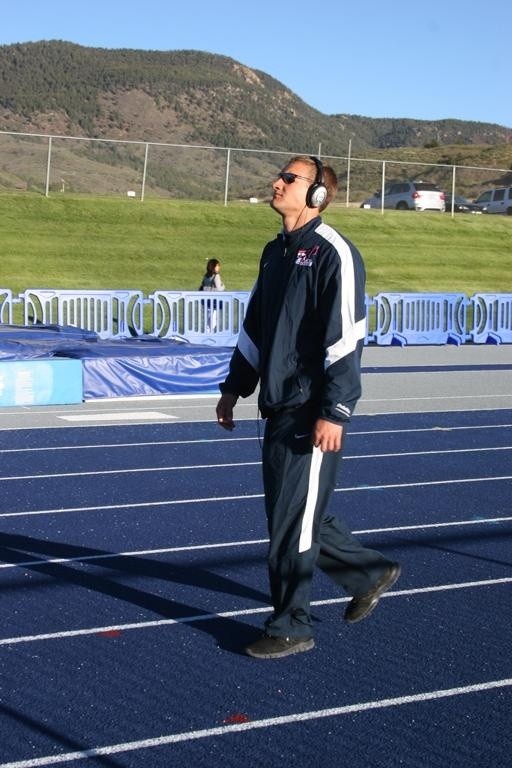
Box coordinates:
[246,634,315,659]
[344,558,401,623]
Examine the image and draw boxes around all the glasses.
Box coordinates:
[277,173,313,184]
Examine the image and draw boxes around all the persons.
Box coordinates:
[197,256,227,332]
[216,157,405,659]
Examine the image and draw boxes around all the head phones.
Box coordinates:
[306,156,328,209]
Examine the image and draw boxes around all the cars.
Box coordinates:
[445,194,488,213]
[471,187,511,215]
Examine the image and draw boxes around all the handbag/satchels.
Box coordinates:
[201,274,223,309]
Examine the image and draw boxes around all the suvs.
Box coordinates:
[360,180,446,211]
